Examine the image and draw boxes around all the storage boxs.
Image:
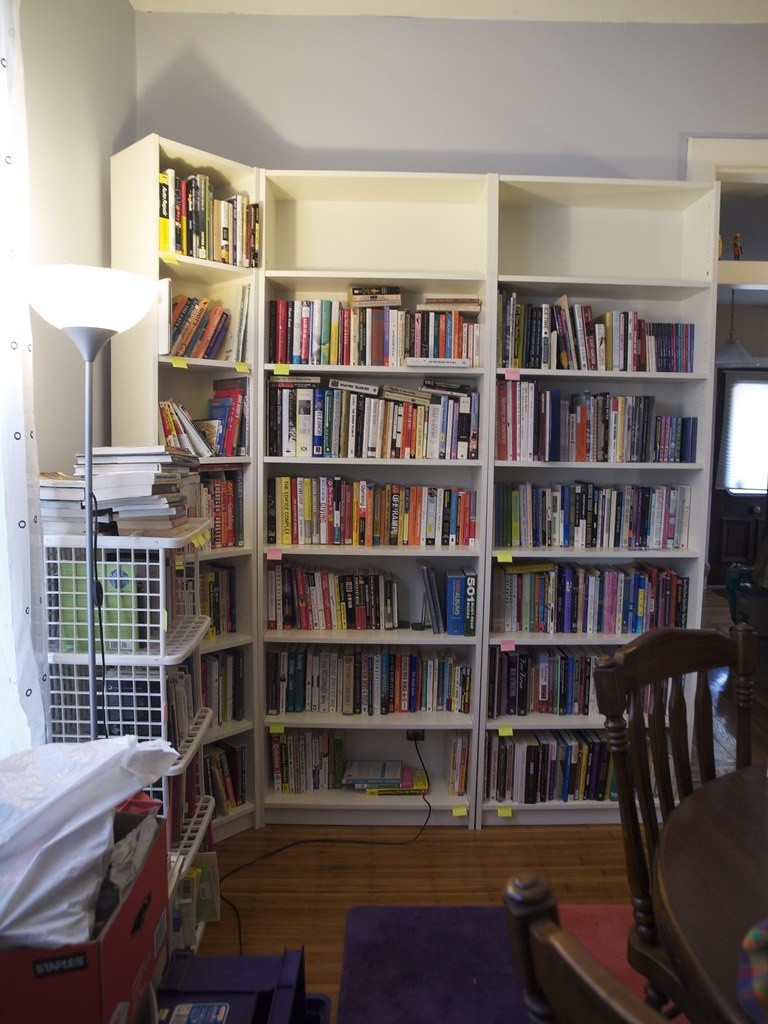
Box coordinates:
[0,812,173,1024]
[145,945,333,1024]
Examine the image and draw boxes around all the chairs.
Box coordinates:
[501,622,757,1024]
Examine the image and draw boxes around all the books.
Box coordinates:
[196,741,247,817]
[55,657,196,752]
[176,564,237,640]
[265,377,697,463]
[158,278,250,362]
[271,731,344,794]
[265,644,684,715]
[158,377,250,457]
[266,559,689,637]
[40,444,199,537]
[269,286,694,373]
[142,755,196,849]
[57,550,175,653]
[171,843,221,949]
[174,465,244,553]
[342,760,428,795]
[267,477,689,547]
[183,648,244,728]
[444,730,675,804]
[159,168,259,270]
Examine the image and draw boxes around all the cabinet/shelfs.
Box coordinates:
[43,519,217,956]
[108,132,721,843]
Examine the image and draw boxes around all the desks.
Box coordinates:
[652,767,768,1024]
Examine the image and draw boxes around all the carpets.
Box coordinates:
[337,904,686,1024]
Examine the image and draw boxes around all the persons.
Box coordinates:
[729,233,743,261]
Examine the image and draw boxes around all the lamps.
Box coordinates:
[21,263,153,742]
